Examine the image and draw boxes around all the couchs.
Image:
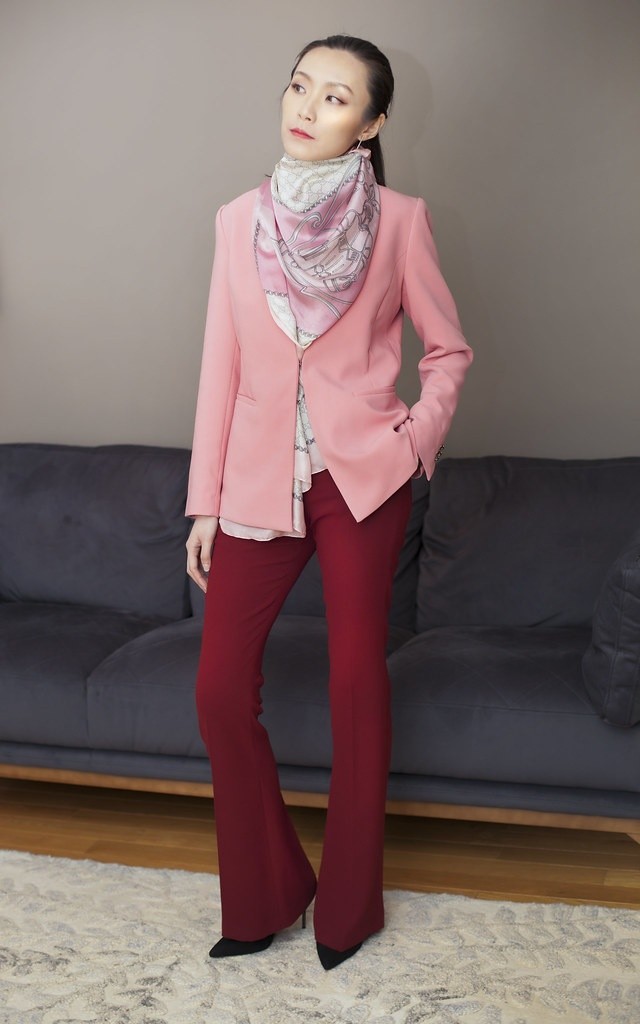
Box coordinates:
[1,444,639,847]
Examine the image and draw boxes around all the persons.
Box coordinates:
[184,32,475,973]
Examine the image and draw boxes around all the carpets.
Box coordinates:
[0,849,639,1024]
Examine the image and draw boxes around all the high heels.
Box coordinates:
[209,886,306,958]
[317,942,363,970]
